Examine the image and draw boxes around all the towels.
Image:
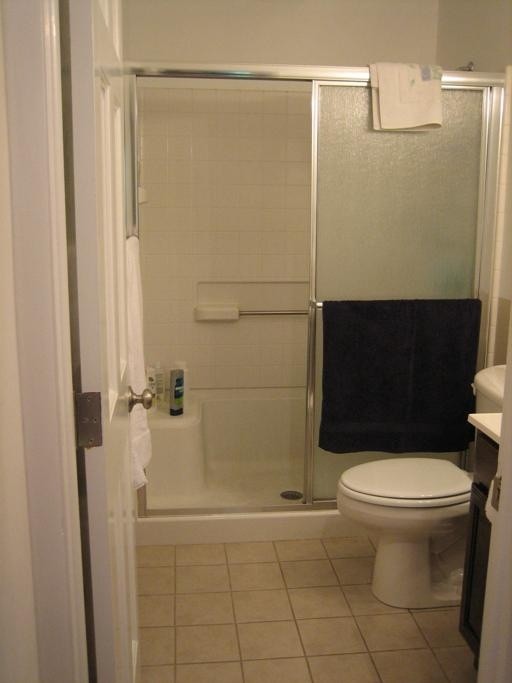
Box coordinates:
[368,60,442,133]
[124,235,154,490]
[315,296,481,454]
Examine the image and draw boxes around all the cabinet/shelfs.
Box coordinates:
[459,429,502,668]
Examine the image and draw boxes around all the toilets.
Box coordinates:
[336,363,507,609]
